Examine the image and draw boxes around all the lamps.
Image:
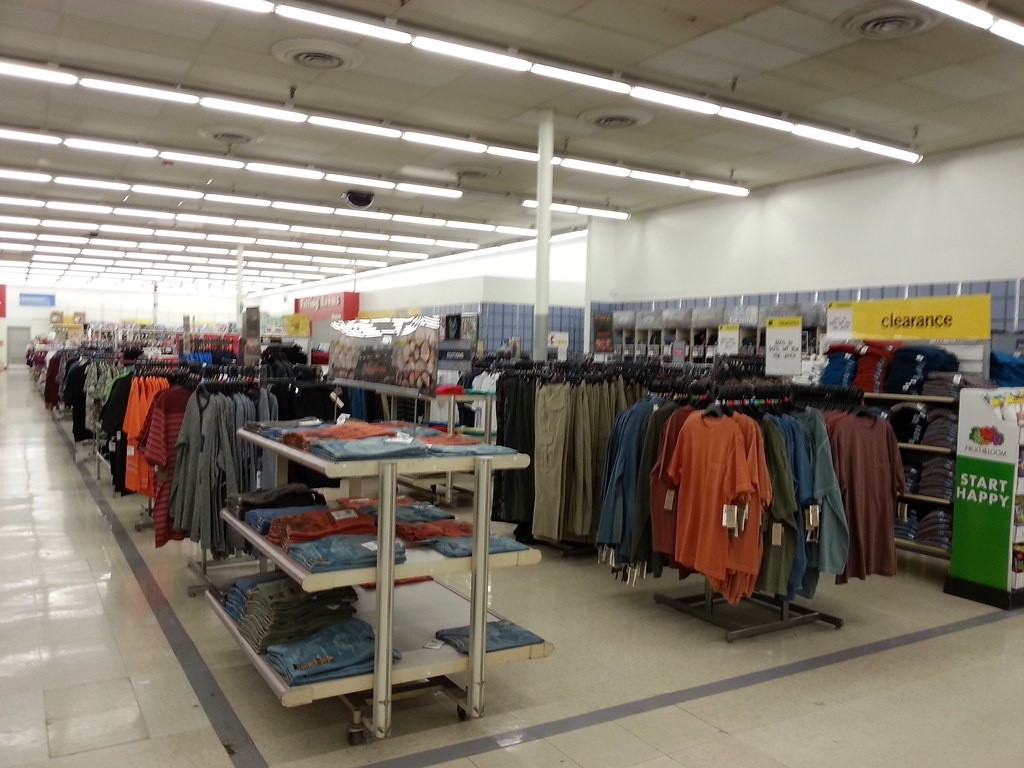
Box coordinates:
[0,0,1024,281]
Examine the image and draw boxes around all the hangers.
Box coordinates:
[472,354,882,419]
[55,339,261,402]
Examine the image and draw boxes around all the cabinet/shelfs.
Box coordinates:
[864,390,955,574]
[331,377,497,508]
[203,427,558,745]
[943,386,1024,612]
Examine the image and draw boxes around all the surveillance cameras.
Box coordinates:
[90,231,99,237]
[344,189,375,210]
